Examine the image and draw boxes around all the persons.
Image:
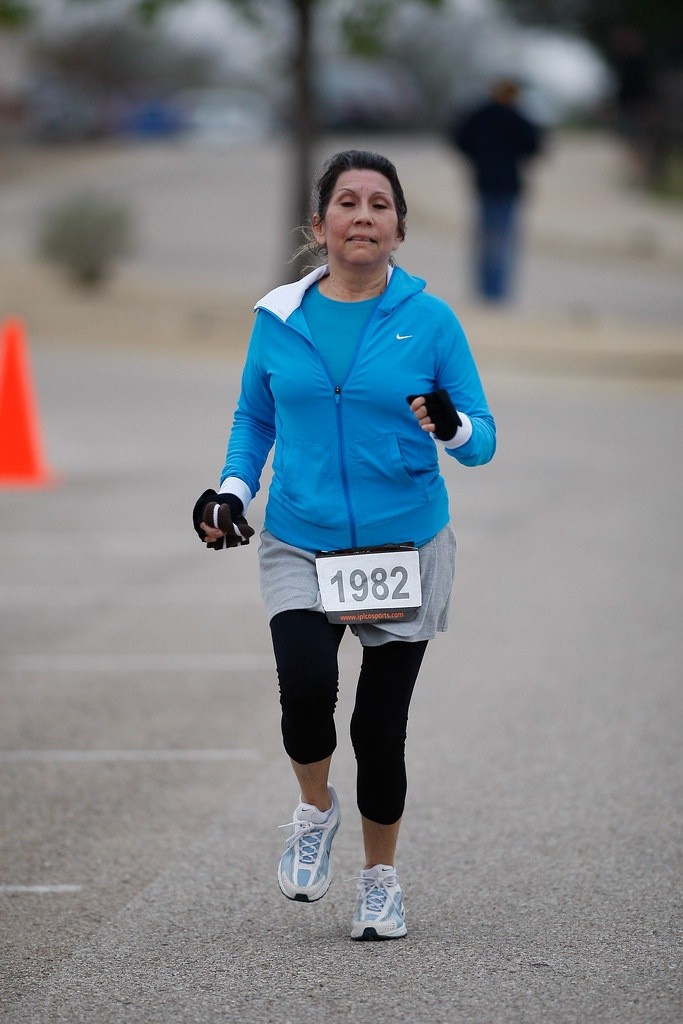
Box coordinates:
[192,149,496,941]
[444,78,549,304]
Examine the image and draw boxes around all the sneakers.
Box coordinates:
[277,782,341,903]
[350,863,408,941]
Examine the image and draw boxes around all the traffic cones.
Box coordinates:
[0,318,60,487]
